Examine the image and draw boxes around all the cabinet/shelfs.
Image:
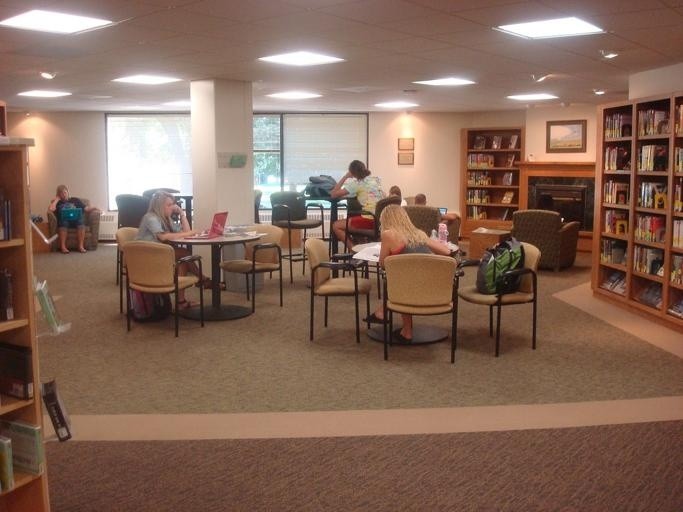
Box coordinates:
[0,136,52,511]
[460,126,525,239]
[590,90,683,334]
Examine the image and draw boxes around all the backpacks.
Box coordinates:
[475,236,526,294]
[304,173,338,198]
[129,288,172,322]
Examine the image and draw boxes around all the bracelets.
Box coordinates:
[180,215,186,217]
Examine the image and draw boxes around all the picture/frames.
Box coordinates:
[398,138,414,150]
[398,153,414,165]
[546,119,587,153]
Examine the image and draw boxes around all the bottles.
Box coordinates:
[430,228,437,241]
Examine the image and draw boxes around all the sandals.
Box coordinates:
[201,280,225,290]
[175,299,202,309]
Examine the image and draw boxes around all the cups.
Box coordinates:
[437,223,449,242]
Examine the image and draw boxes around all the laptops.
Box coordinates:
[58,208,82,227]
[439,208,448,216]
[184,211,228,241]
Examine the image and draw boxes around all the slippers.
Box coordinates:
[362,311,391,325]
[393,326,413,345]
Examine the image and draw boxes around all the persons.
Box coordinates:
[389,185,407,206]
[330,160,386,252]
[362,204,452,345]
[48,184,101,254]
[415,194,426,205]
[134,191,226,310]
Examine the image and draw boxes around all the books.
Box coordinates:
[466,135,495,221]
[505,153,515,168]
[600,104,683,319]
[0,416,45,477]
[501,191,514,204]
[36,280,62,337]
[0,268,15,321]
[502,208,510,221]
[502,172,512,186]
[0,199,13,241]
[492,135,503,150]
[0,436,13,492]
[42,380,72,442]
[0,341,34,400]
[508,135,518,149]
